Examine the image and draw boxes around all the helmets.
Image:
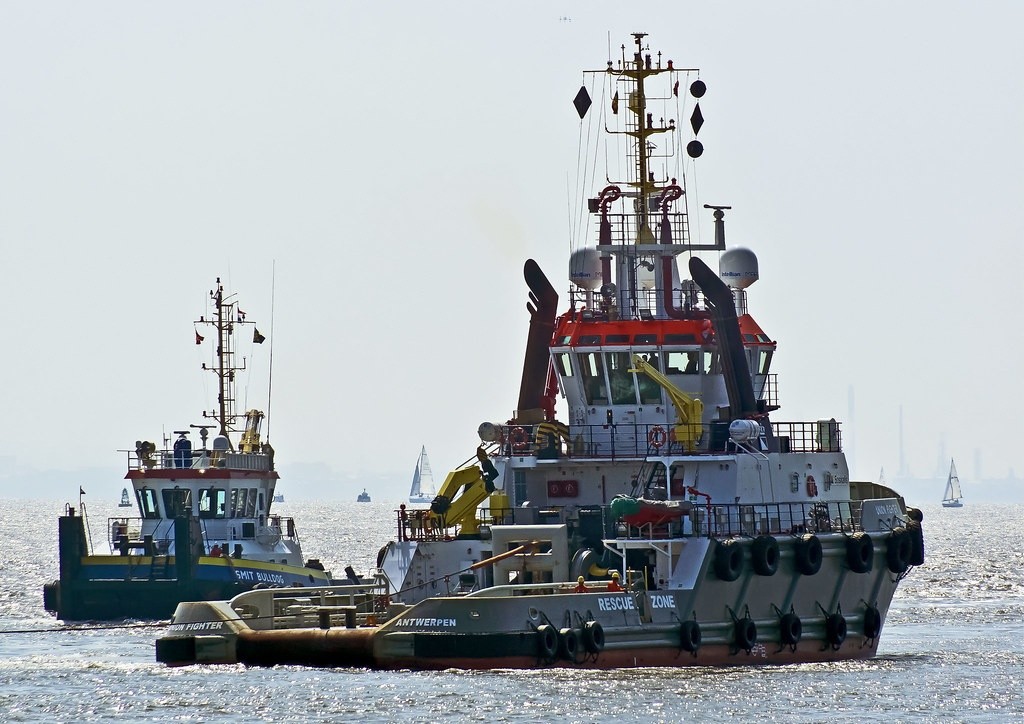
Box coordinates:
[612,572,620,578]
[578,576,584,582]
[214,542,218,545]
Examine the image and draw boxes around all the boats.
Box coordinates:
[118,489,133,507]
[274,493,285,504]
[156,19,924,673]
[357,488,370,503]
[45,276,331,621]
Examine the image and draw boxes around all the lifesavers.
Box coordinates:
[781,612,803,643]
[583,621,606,652]
[424,517,433,534]
[509,426,528,449]
[849,532,873,573]
[753,535,780,576]
[680,620,702,650]
[718,541,745,581]
[807,475,817,496]
[829,612,847,642]
[536,624,558,657]
[888,528,912,573]
[863,608,882,639]
[908,520,925,565]
[796,534,823,574]
[735,617,758,649]
[649,427,666,447]
[559,628,579,658]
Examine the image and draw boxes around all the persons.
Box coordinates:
[607,573,624,592]
[574,576,589,593]
[642,353,658,372]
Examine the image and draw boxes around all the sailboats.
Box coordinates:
[409,445,437,504]
[942,458,965,507]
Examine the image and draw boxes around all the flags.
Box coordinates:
[196,331,203,344]
[253,328,265,344]
